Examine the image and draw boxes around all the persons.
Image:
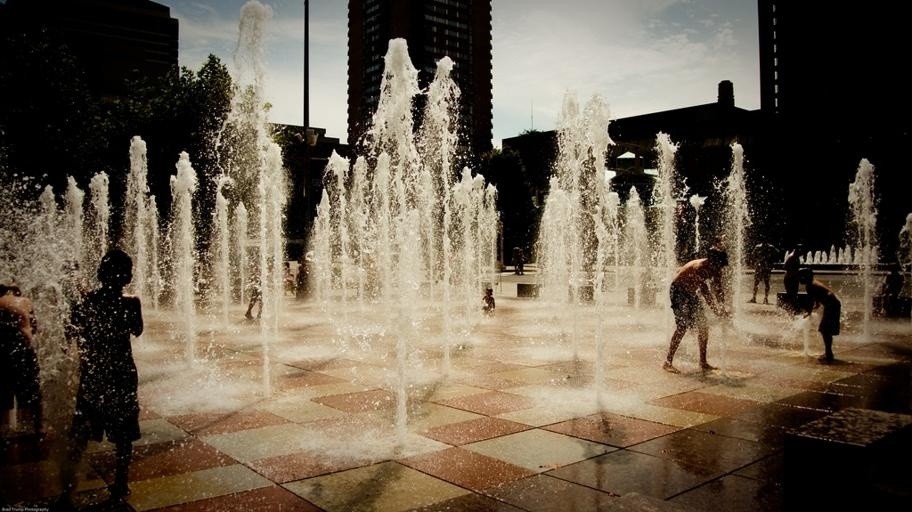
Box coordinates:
[480,287,497,312]
[0,281,50,464]
[879,262,908,319]
[746,230,783,304]
[660,245,731,375]
[890,262,912,318]
[57,246,144,511]
[56,259,97,357]
[517,249,525,276]
[242,285,265,323]
[26,280,63,319]
[512,245,520,276]
[796,265,843,363]
[779,240,809,314]
